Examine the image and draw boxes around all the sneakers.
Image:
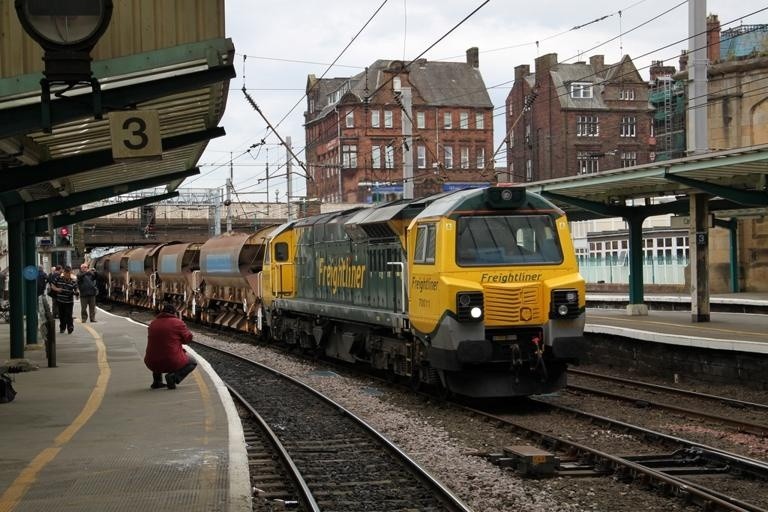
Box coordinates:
[54,315,98,334]
[150,373,176,390]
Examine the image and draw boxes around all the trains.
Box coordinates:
[83,183,595,412]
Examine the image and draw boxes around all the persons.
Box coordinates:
[145,304,197,389]
[38,264,100,333]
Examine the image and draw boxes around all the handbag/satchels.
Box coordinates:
[47,285,56,298]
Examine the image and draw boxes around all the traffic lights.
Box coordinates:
[55,226,75,237]
